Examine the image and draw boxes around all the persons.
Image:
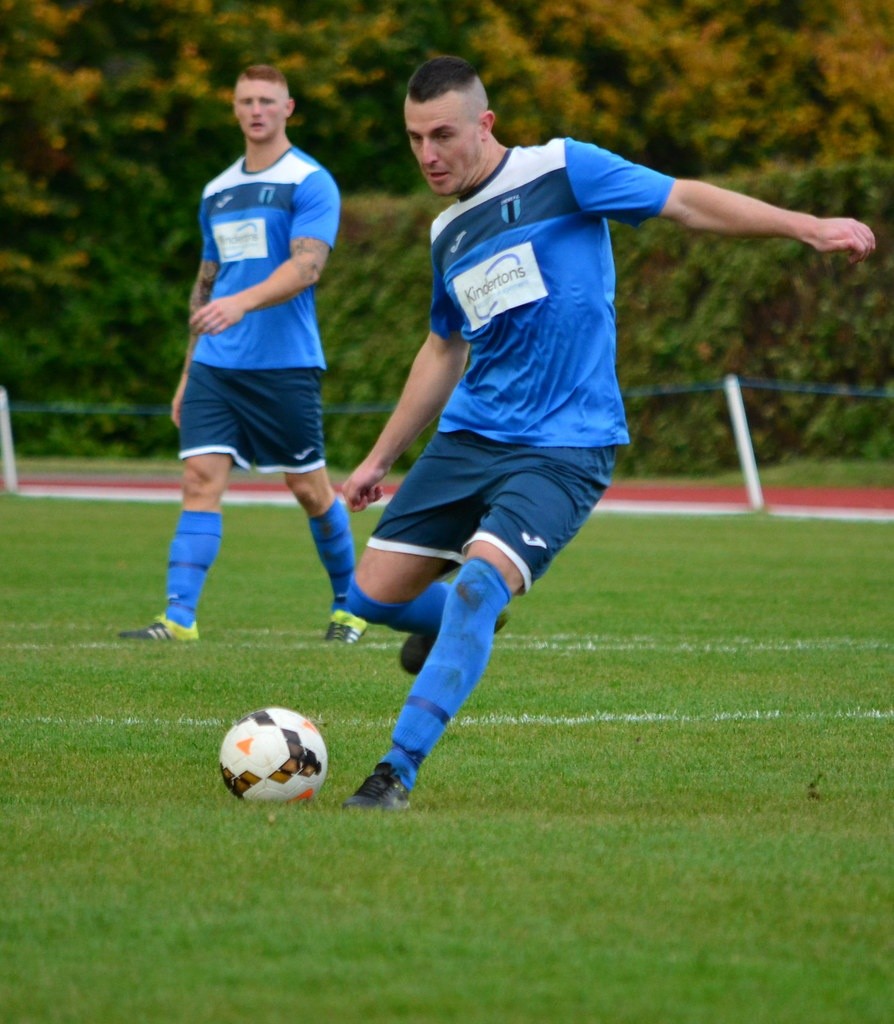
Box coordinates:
[340,57,877,808]
[116,65,371,643]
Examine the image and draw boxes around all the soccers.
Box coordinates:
[218,706,330,805]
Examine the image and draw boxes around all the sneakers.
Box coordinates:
[118,613,200,643]
[401,605,510,673]
[325,600,366,645]
[340,762,410,812]
